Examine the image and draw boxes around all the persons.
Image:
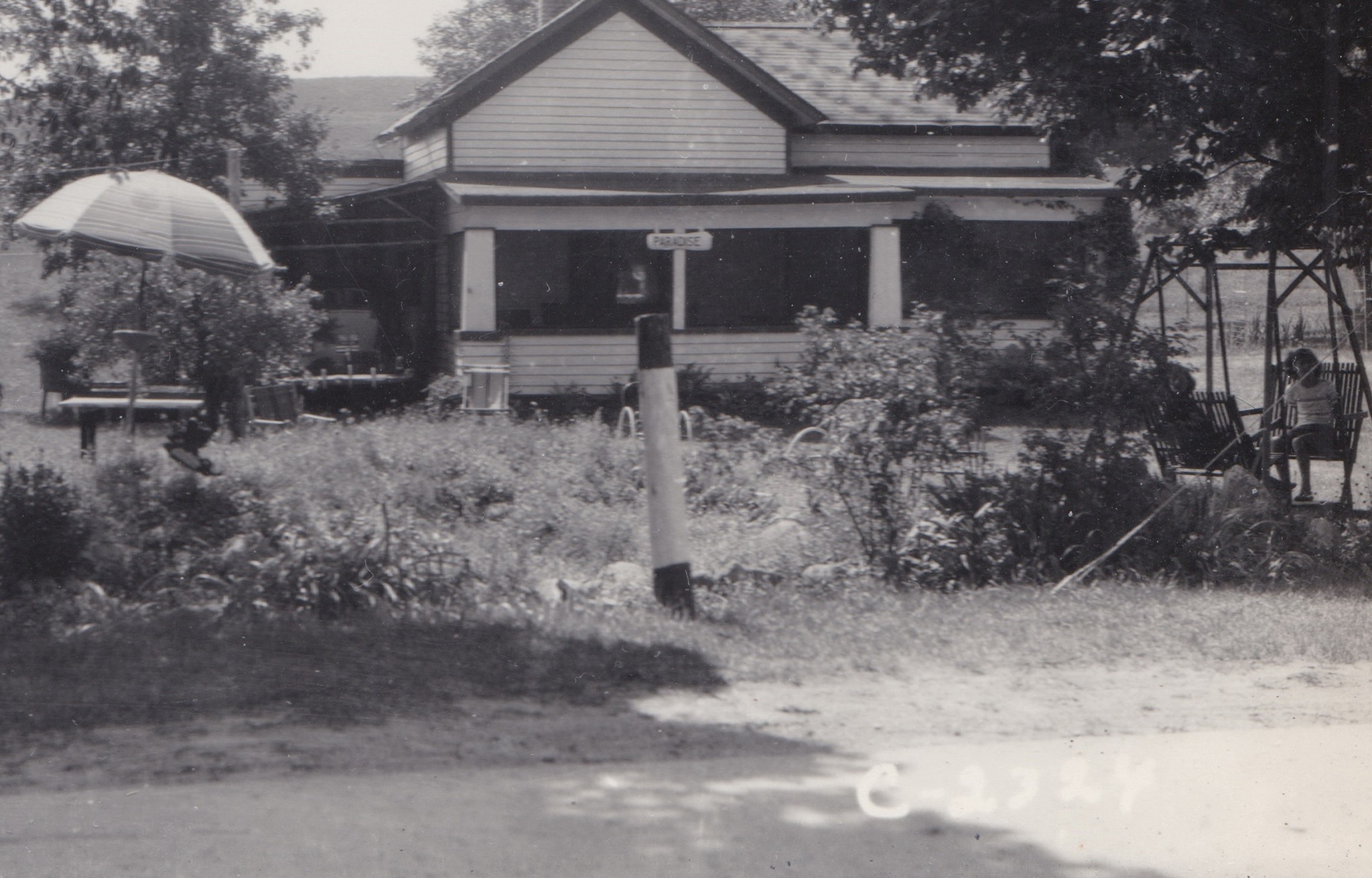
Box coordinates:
[1165,367,1297,493]
[12,169,277,434]
[1271,348,1347,501]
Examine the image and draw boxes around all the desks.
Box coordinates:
[58,394,199,461]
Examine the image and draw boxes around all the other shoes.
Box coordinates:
[1288,483,1296,489]
[1295,493,1314,500]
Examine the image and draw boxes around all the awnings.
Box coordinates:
[242,174,433,217]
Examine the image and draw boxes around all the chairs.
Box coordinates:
[244,383,335,438]
[1259,362,1365,514]
[1141,389,1294,519]
